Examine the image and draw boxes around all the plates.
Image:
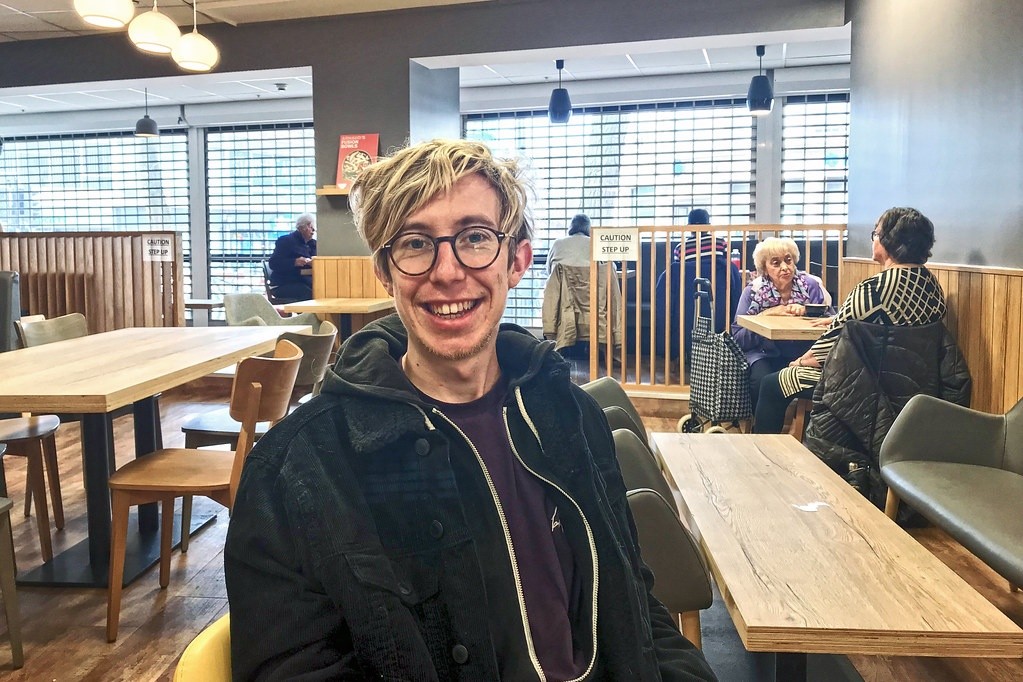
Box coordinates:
[342,150,373,181]
[802,317,818,320]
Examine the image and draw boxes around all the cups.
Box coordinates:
[338,184,347,189]
[805,304,829,317]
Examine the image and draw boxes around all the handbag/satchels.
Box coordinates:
[841,464,871,506]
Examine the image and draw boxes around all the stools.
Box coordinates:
[0,496,24,668]
[0,414,68,567]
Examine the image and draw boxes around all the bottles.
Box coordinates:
[730,248,740,271]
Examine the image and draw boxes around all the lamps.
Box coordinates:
[545,58,571,125]
[745,43,775,115]
[73,0,231,73]
[134,87,160,138]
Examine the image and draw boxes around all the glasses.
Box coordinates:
[375,228,519,278]
[870,230,882,242]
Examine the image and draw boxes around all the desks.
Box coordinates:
[736,311,834,345]
[183,298,225,310]
[283,297,396,346]
[647,425,1023,681]
[0,322,315,590]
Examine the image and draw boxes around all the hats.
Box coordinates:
[687,209,710,226]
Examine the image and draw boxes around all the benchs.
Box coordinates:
[606,238,847,342]
[876,392,1023,603]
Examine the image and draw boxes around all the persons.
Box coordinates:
[752,207,946,434]
[223,141,720,682]
[674,210,731,266]
[269,214,319,301]
[546,214,620,274]
[730,237,836,435]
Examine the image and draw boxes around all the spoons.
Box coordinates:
[825,312,831,317]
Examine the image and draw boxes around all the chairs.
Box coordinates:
[222,293,323,341]
[568,375,711,663]
[181,319,341,555]
[99,340,303,645]
[12,313,167,519]
[261,259,305,304]
